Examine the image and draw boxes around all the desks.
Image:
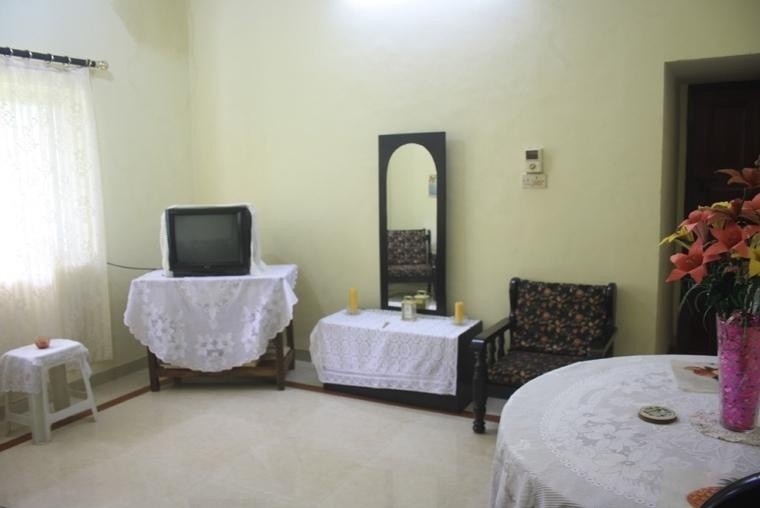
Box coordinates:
[319,307,482,416]
[132,265,301,390]
[496,350,760,508]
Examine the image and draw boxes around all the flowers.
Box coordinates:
[658,149,760,350]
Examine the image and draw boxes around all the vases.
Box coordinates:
[716,312,759,431]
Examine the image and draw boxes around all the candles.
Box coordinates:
[455,302,464,324]
[349,288,358,314]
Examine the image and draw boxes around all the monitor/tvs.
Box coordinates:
[166,206,252,277]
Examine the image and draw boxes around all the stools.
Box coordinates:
[3,332,99,447]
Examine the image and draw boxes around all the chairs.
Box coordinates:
[473,273,620,435]
[387,227,437,294]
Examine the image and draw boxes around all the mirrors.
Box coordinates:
[374,132,449,317]
